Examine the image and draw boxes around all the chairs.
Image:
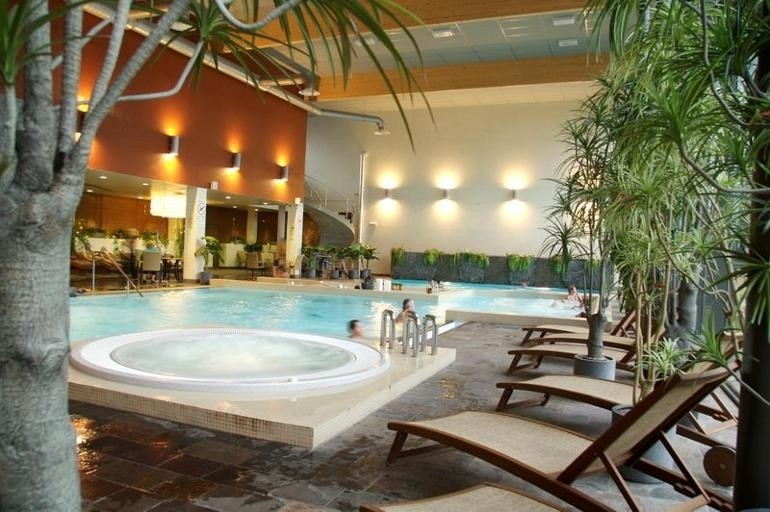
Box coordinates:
[70,249,184,284]
[497,330,740,487]
[238,252,305,279]
[506,306,744,377]
[386,359,741,511]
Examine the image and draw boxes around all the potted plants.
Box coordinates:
[302,241,379,279]
[543,70,669,383]
[195,236,225,285]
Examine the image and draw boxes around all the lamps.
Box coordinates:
[384,188,517,200]
[353,15,578,47]
[169,135,288,181]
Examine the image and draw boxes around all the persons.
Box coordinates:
[430,279,446,288]
[273,258,286,277]
[335,257,348,278]
[396,298,419,325]
[129,236,169,284]
[348,319,363,338]
[72,224,123,272]
[563,285,582,303]
